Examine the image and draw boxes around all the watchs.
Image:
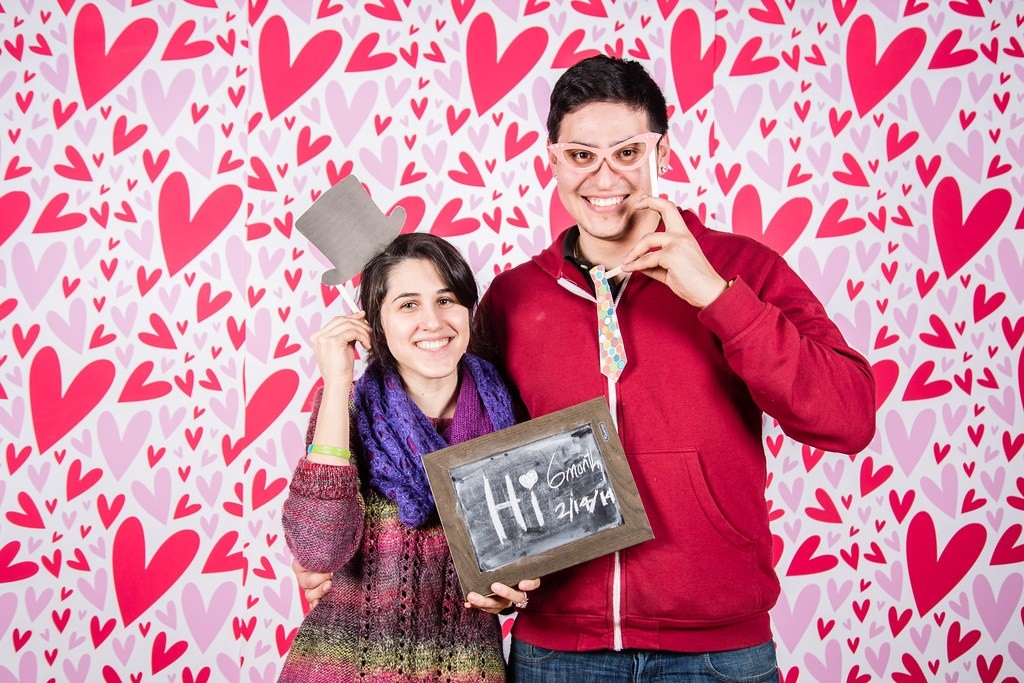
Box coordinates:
[727,278,734,288]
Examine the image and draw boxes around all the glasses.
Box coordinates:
[546,132,665,174]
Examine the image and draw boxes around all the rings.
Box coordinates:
[515,590,529,609]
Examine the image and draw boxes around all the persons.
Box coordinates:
[290,51,877,683]
[277,233,541,683]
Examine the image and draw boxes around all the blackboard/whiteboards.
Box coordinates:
[420,391,654,604]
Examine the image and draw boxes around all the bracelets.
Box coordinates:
[306,443,351,461]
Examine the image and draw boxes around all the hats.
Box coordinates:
[292,175,406,286]
